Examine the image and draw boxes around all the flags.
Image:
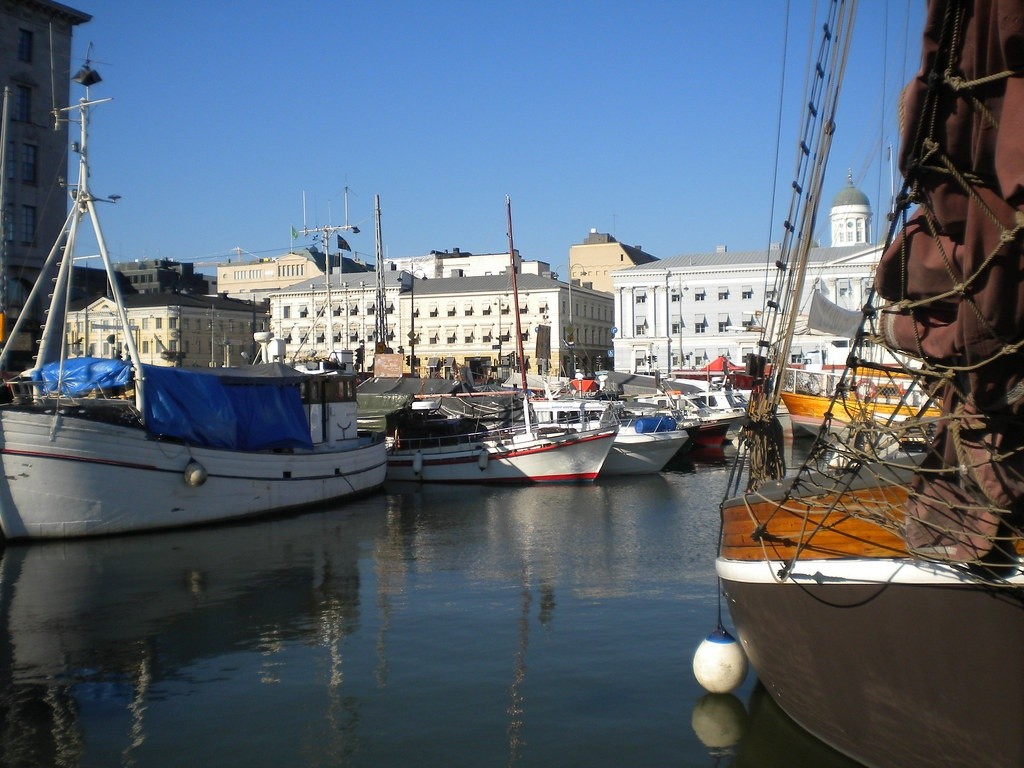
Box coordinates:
[292,226,298,239]
[337,235,352,252]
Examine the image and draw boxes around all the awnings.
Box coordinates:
[425,357,440,368]
[500,356,510,365]
[414,302,546,339]
[443,357,455,367]
[536,357,548,365]
[632,286,753,359]
[282,303,393,339]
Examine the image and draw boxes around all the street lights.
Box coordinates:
[396,263,429,378]
[551,262,589,378]
[204,304,221,367]
[493,294,505,367]
[505,285,530,390]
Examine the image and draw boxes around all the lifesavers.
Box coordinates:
[856,378,877,400]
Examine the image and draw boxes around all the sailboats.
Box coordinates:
[711,1,1024,768]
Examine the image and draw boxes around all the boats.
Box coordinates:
[0,21,388,543]
[354,191,948,485]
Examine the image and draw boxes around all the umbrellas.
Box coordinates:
[699,355,744,371]
